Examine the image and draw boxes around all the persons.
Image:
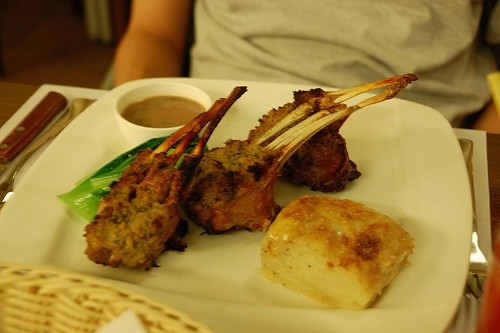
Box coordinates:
[109,0,500,134]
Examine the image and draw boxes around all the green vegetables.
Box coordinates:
[57,133,210,223]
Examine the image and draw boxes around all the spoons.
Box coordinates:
[0,98,96,210]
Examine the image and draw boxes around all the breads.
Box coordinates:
[259,195,415,308]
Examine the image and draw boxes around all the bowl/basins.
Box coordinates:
[115,83,212,145]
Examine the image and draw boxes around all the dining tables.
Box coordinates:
[0,82,500,332]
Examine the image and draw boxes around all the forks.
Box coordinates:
[458,139,490,303]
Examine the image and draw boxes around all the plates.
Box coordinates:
[0,77,474,333]
